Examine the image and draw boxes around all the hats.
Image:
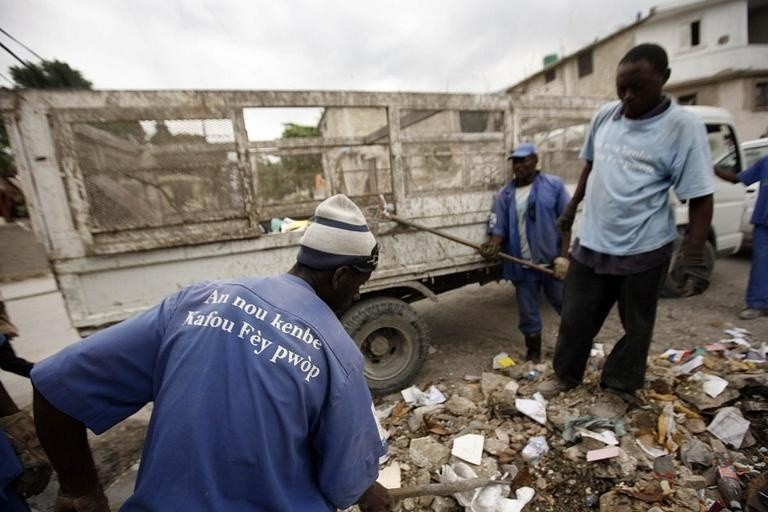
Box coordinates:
[507,142,538,161]
[296,193,378,273]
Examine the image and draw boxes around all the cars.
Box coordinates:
[713,137,768,243]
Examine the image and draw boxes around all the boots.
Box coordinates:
[524,330,542,365]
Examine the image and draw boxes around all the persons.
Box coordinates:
[534,43,716,423]
[478,143,576,365]
[0,428,54,511]
[29,194,396,512]
[1,330,37,380]
[714,156,768,321]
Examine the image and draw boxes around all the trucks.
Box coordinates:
[0,87,745,399]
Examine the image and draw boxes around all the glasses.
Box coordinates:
[330,243,379,273]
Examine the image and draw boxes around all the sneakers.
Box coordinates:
[740,305,768,319]
[536,372,569,398]
[588,392,630,423]
